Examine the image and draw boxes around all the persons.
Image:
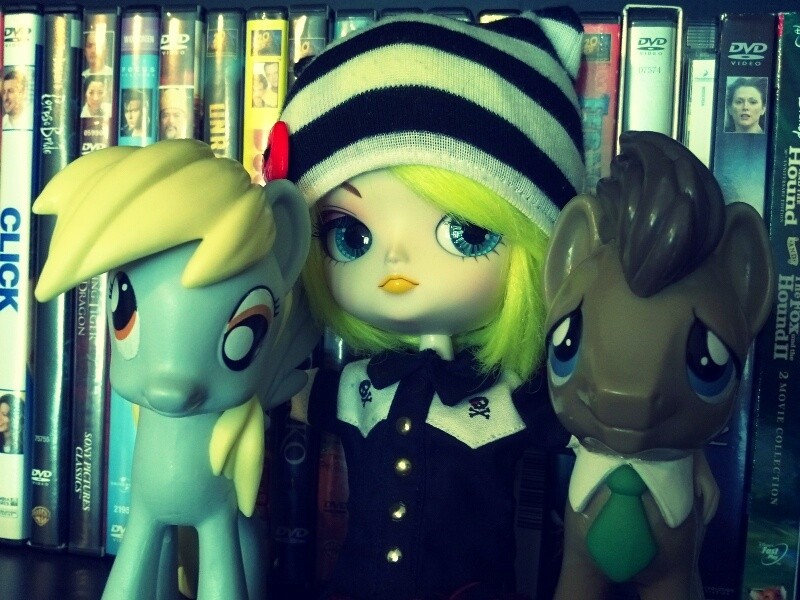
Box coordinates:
[3,70,33,127]
[254,74,268,108]
[263,62,278,108]
[125,91,148,136]
[80,75,111,116]
[726,77,767,134]
[83,33,112,75]
[0,394,25,454]
[160,89,185,138]
[263,13,722,600]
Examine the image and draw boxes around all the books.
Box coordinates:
[576,5,800,600]
[0,9,376,555]
